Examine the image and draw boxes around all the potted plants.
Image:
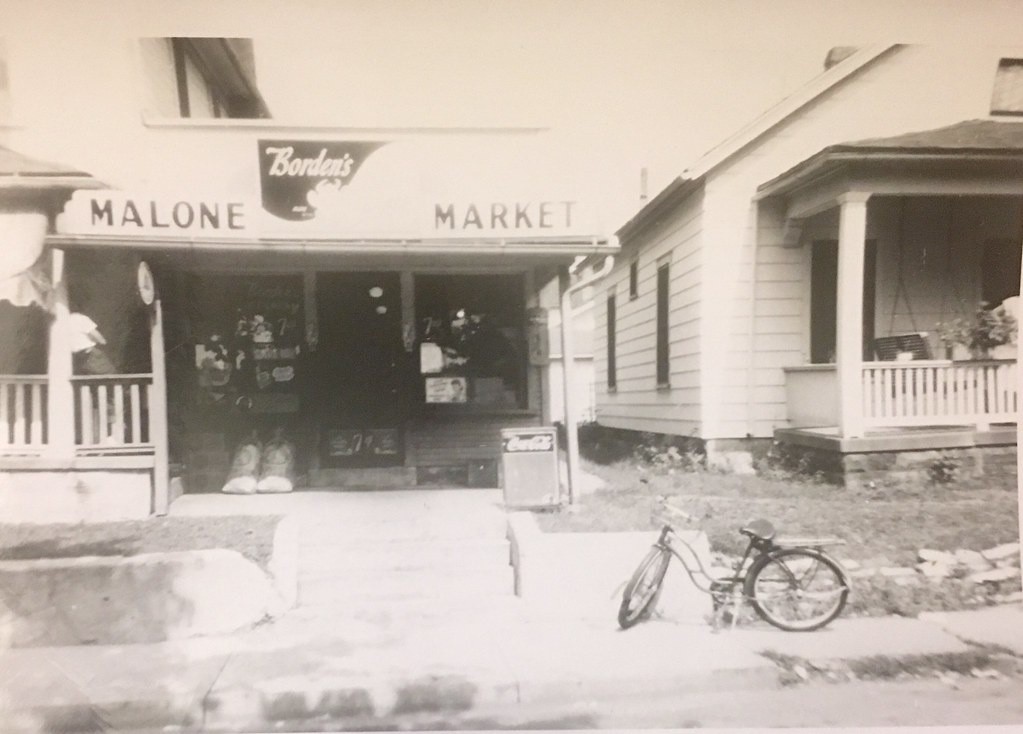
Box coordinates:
[939,303,1015,361]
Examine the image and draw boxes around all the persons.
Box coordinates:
[68,295,126,404]
[449,380,466,401]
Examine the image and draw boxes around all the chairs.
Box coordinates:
[873,334,930,361]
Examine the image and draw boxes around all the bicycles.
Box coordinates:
[618,494,852,632]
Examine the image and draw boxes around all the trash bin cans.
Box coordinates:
[499,426,562,511]
